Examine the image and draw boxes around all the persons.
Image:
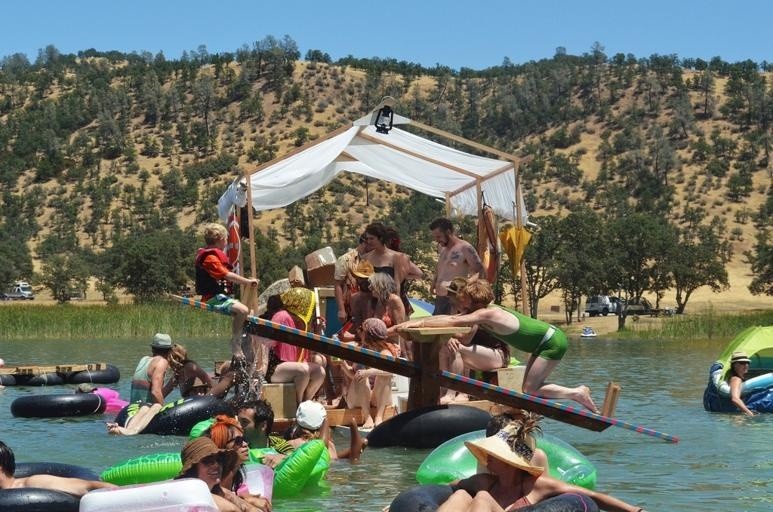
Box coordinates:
[387,279,602,416]
[235,400,295,469]
[285,399,338,459]
[382,423,650,512]
[172,437,263,512]
[727,352,753,415]
[194,223,260,363]
[131,333,173,407]
[161,343,214,408]
[259,217,509,435]
[0,441,119,498]
[200,415,272,512]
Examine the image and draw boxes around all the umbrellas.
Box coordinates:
[497,205,532,281]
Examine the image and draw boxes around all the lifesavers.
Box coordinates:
[10,393,108,418]
[138,393,236,436]
[365,403,494,445]
[390,428,599,512]
[0,365,118,384]
[104,444,330,499]
[0,460,103,512]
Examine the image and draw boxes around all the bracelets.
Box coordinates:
[631,506,642,512]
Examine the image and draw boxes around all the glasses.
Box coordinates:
[227,435,248,445]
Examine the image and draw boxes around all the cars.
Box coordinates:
[2,281,34,302]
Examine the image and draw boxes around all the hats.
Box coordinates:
[362,318,389,339]
[464,408,545,479]
[174,437,238,479]
[296,399,327,429]
[348,259,375,280]
[279,287,315,323]
[148,333,174,351]
[730,352,752,362]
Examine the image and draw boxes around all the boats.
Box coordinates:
[211,95,532,451]
[701,324,772,417]
[0,362,121,386]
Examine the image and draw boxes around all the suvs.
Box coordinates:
[584,295,623,317]
